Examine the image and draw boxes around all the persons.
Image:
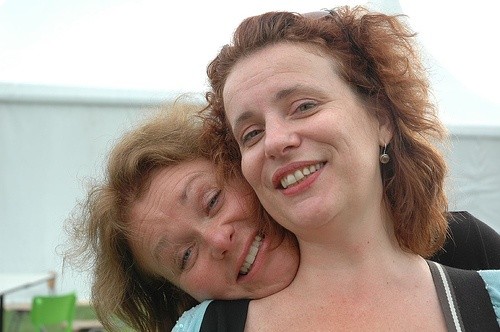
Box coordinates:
[65,92,500,332]
[172,6,500,332]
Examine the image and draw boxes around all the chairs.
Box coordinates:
[24,293,76,332]
